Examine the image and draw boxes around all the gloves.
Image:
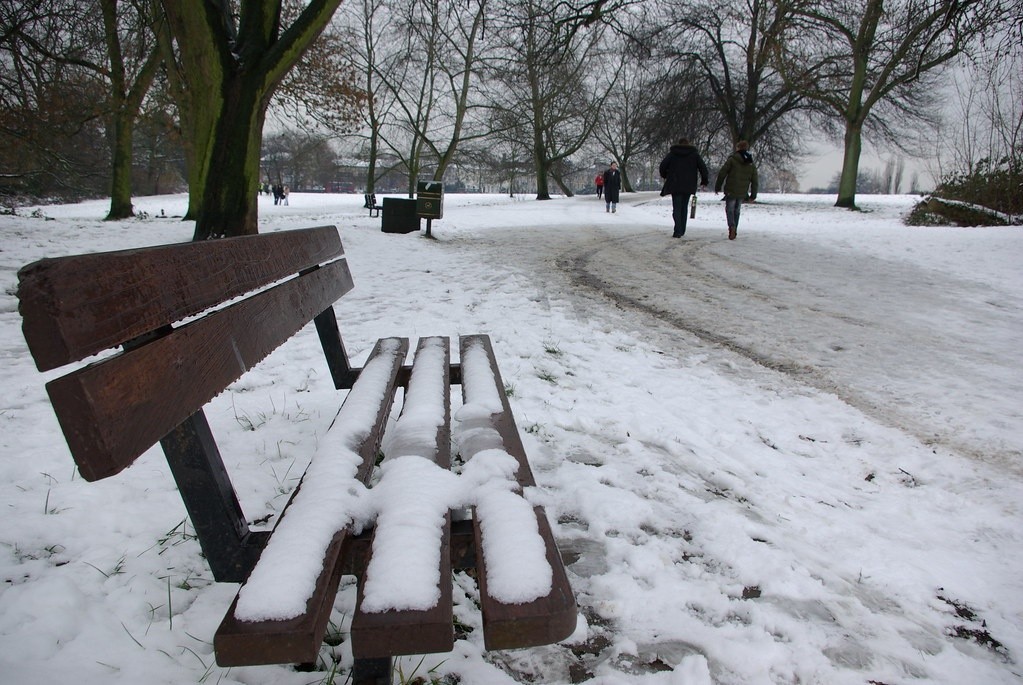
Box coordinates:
[748,195,756,202]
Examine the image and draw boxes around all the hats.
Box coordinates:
[736,141,749,150]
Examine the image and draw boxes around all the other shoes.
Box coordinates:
[729,226,736,240]
[607,209,609,211]
[673,232,683,238]
[612,210,616,213]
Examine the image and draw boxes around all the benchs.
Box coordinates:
[364,193,382,218]
[18,225,578,684]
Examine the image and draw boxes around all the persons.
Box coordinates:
[714,140,758,241]
[659,137,709,238]
[272,184,289,206]
[595,161,620,213]
